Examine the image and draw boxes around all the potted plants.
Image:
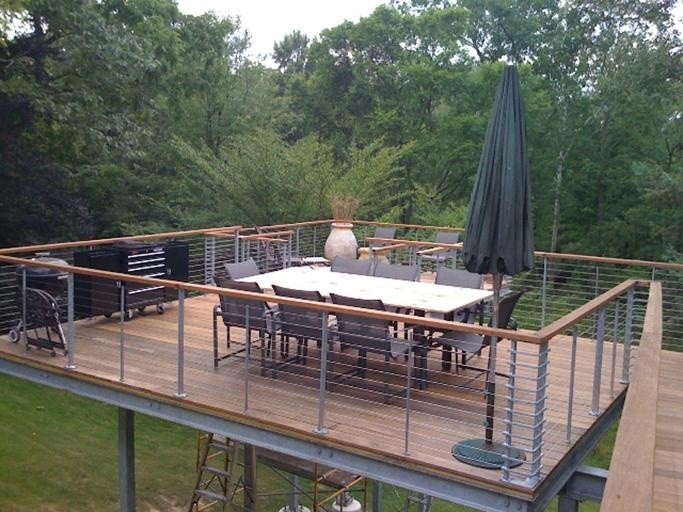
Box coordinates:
[323,193,361,262]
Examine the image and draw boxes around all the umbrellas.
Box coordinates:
[460,50,538,442]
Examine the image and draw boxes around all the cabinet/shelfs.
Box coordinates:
[72,240,189,322]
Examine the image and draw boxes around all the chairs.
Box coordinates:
[223,256,284,356]
[434,291,523,401]
[328,255,372,276]
[373,264,422,314]
[271,285,362,390]
[329,293,422,404]
[213,277,302,378]
[427,268,483,369]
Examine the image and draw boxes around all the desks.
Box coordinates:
[235,267,494,390]
